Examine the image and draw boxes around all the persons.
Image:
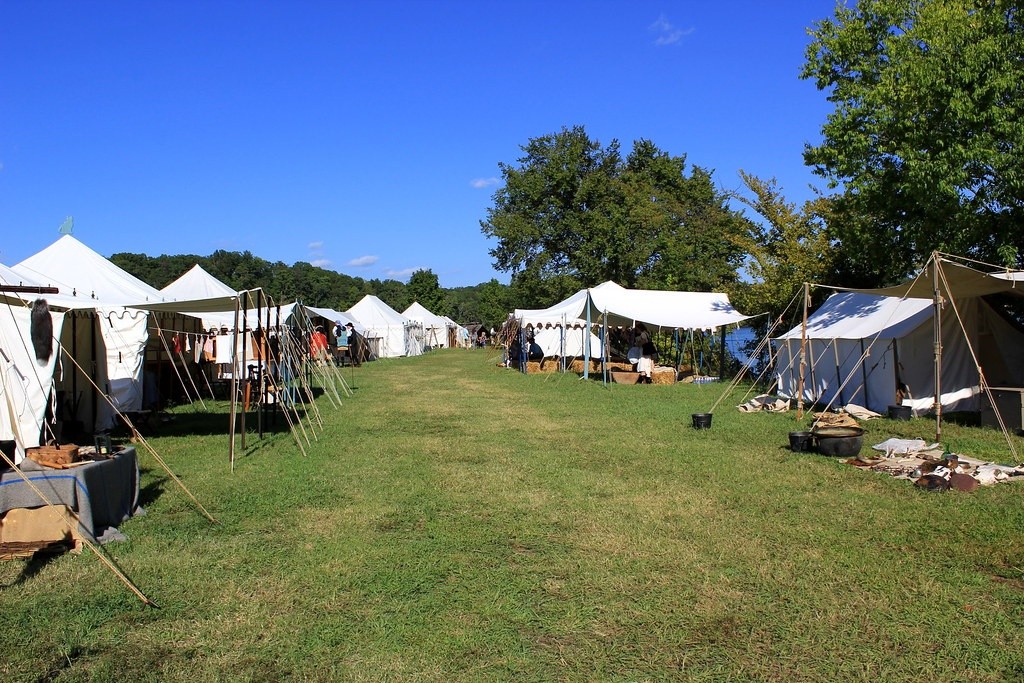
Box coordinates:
[463,327,486,350]
[527,337,544,360]
[633,324,653,384]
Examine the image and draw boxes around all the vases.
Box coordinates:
[788,431,814,453]
[886,406,912,421]
[692,412,711,430]
[812,425,865,458]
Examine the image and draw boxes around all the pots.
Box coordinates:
[693,413,713,430]
[810,425,868,458]
[788,432,814,452]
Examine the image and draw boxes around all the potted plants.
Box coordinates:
[941,443,959,469]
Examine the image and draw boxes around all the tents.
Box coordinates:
[1,233,238,465]
[777,291,1023,420]
[345,293,465,358]
[501,279,626,359]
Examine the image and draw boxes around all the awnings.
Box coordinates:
[803,249,1024,443]
[577,287,770,382]
[122,286,385,460]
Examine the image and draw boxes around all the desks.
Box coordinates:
[0,445,143,554]
